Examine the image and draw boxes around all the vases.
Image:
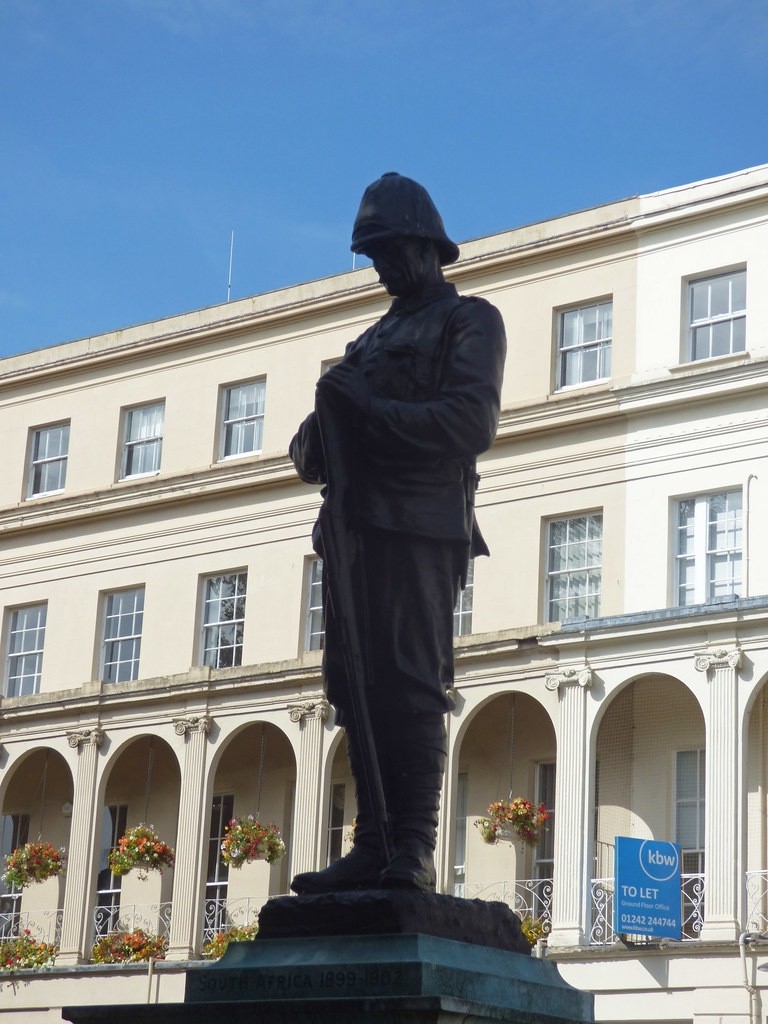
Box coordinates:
[495,820,520,842]
[245,841,269,860]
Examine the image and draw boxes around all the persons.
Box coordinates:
[290,172,507,894]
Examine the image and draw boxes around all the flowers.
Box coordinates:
[205,926,259,961]
[472,797,549,854]
[522,916,542,944]
[221,814,286,870]
[89,924,166,965]
[1,834,66,890]
[0,929,57,973]
[107,823,175,882]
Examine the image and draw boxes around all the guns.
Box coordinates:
[316,389,401,888]
